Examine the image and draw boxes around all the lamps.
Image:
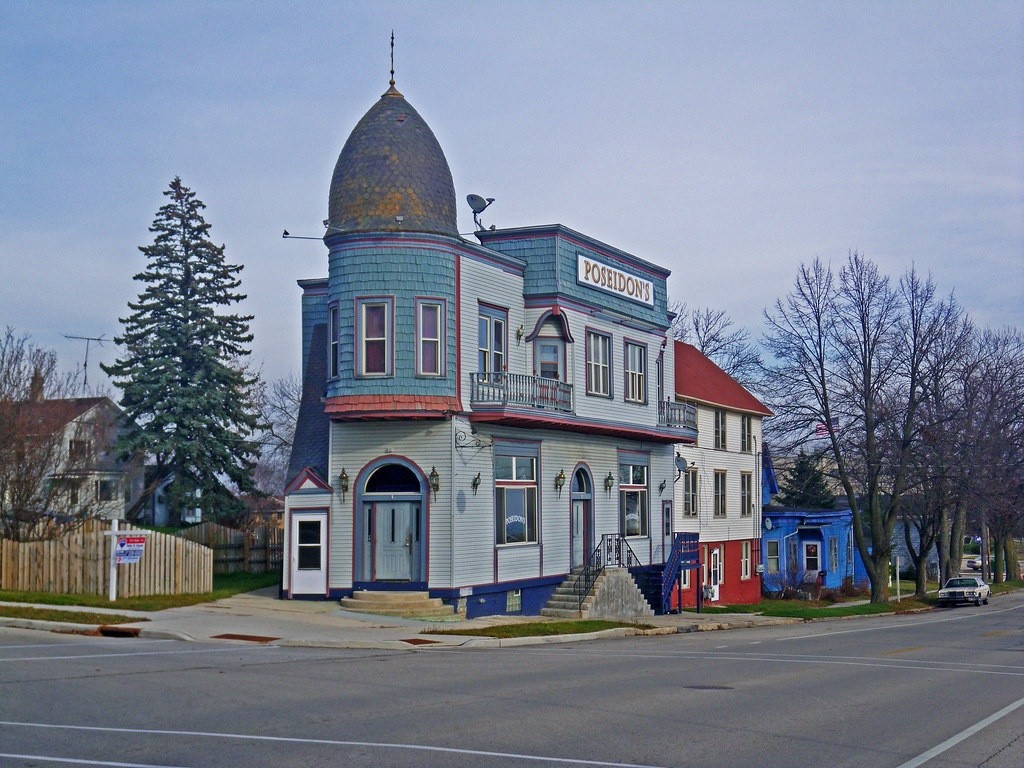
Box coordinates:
[658,479,666,496]
[430,465,441,501]
[555,468,565,501]
[604,471,614,498]
[472,472,482,495]
[339,466,349,502]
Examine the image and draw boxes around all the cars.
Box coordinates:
[966,557,996,572]
[937,578,990,606]
